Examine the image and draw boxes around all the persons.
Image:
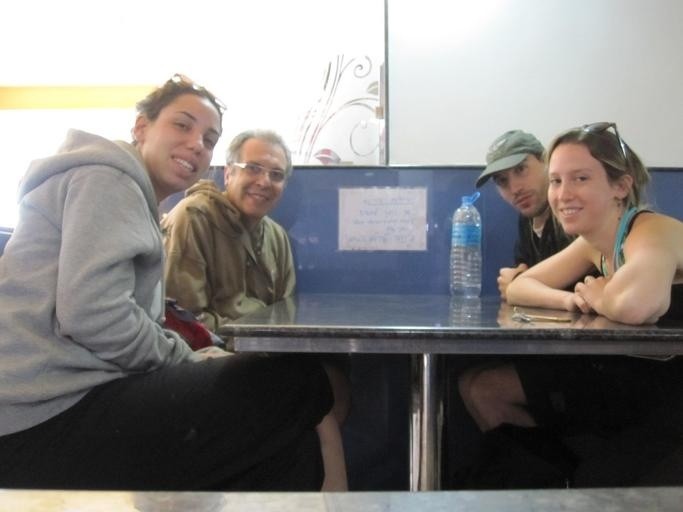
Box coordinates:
[0,70,330,491]
[456,128,602,487]
[158,130,354,490]
[504,121,682,488]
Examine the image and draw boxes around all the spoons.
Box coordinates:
[512,304,575,324]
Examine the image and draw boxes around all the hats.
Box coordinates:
[475,128,546,190]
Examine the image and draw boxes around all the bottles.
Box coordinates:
[448,190,487,302]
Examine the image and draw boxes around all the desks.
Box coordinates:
[216,292,682,494]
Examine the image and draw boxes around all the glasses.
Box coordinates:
[576,121,629,165]
[163,72,230,117]
[234,161,288,183]
